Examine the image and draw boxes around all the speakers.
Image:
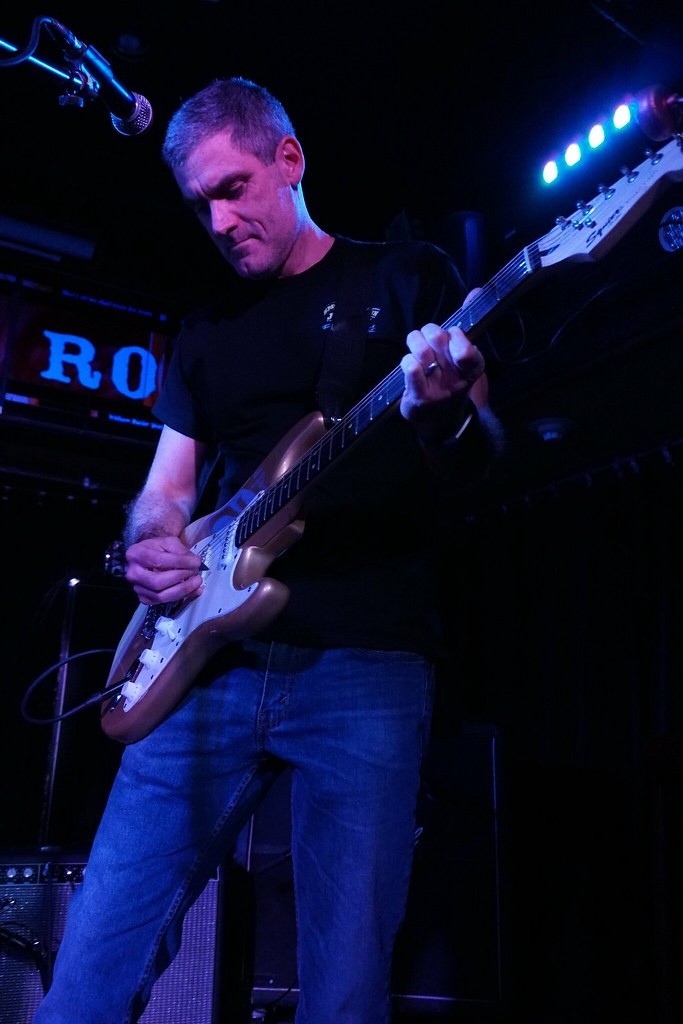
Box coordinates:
[0,854,259,1024]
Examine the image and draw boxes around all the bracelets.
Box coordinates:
[416,397,477,448]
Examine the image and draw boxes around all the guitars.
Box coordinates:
[97,133,683,750]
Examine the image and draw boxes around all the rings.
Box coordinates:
[423,360,439,377]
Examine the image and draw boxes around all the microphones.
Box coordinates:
[41,17,153,136]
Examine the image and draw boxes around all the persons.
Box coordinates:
[27,74,505,1024]
